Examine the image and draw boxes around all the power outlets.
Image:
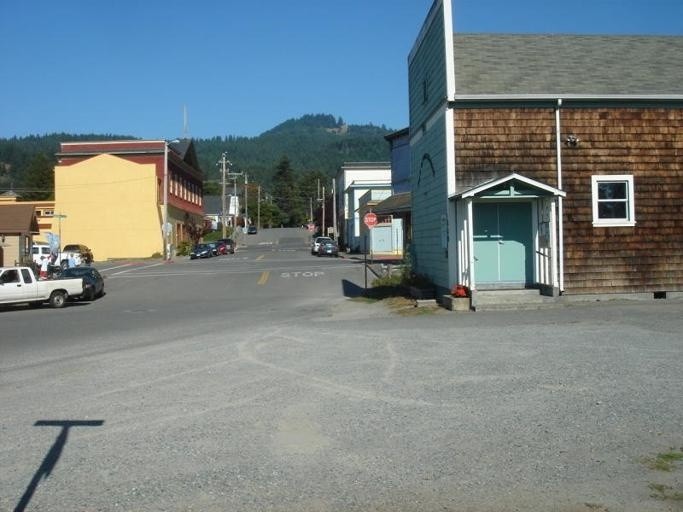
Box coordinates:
[32,245,80,272]
[0,267,83,308]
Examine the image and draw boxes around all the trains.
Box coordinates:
[364,213,378,228]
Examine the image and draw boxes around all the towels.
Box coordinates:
[346,249,351,254]
[242,228,248,234]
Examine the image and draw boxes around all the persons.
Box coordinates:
[48,243,58,265]
[74,255,81,268]
[68,253,75,269]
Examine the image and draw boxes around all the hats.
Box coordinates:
[60,267,104,301]
[247,226,256,235]
[63,244,93,265]
[318,241,338,257]
[189,238,235,259]
[311,237,333,256]
